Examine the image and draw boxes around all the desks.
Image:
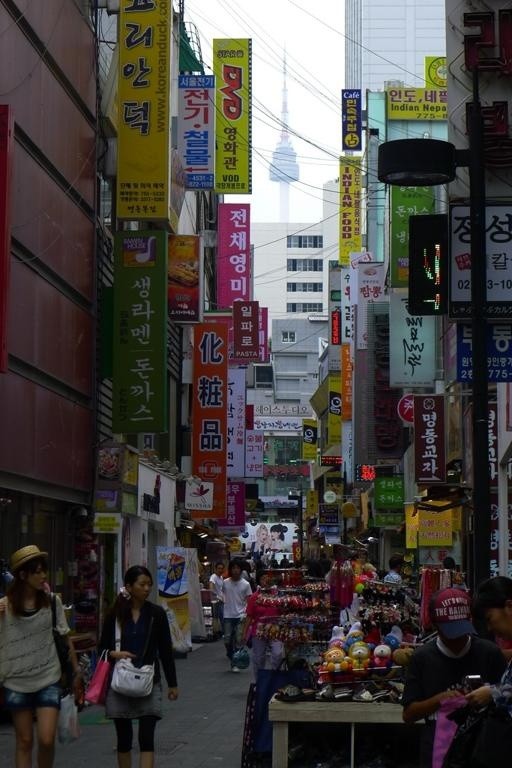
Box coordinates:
[70,632,98,670]
[268,688,438,768]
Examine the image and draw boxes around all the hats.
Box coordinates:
[10,544,49,571]
[429,587,479,640]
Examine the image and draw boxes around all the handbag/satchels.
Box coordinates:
[57,692,81,745]
[230,644,250,670]
[111,657,156,698]
[49,592,70,667]
[83,649,112,705]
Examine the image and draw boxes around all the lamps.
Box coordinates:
[445,459,464,479]
[138,454,202,486]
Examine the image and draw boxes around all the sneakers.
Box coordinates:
[231,666,240,673]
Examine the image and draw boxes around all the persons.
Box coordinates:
[221,558,254,673]
[398,587,507,766]
[208,560,226,633]
[440,556,465,588]
[382,554,407,583]
[464,576,512,734]
[96,562,179,767]
[245,568,289,683]
[241,549,332,588]
[0,542,84,767]
[270,523,292,551]
[249,522,270,558]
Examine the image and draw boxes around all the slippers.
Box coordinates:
[315,684,354,702]
[352,687,390,703]
[275,683,317,702]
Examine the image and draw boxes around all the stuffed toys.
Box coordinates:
[321,647,353,675]
[369,625,407,667]
[344,620,365,651]
[343,641,371,669]
[325,624,346,650]
[369,640,396,674]
